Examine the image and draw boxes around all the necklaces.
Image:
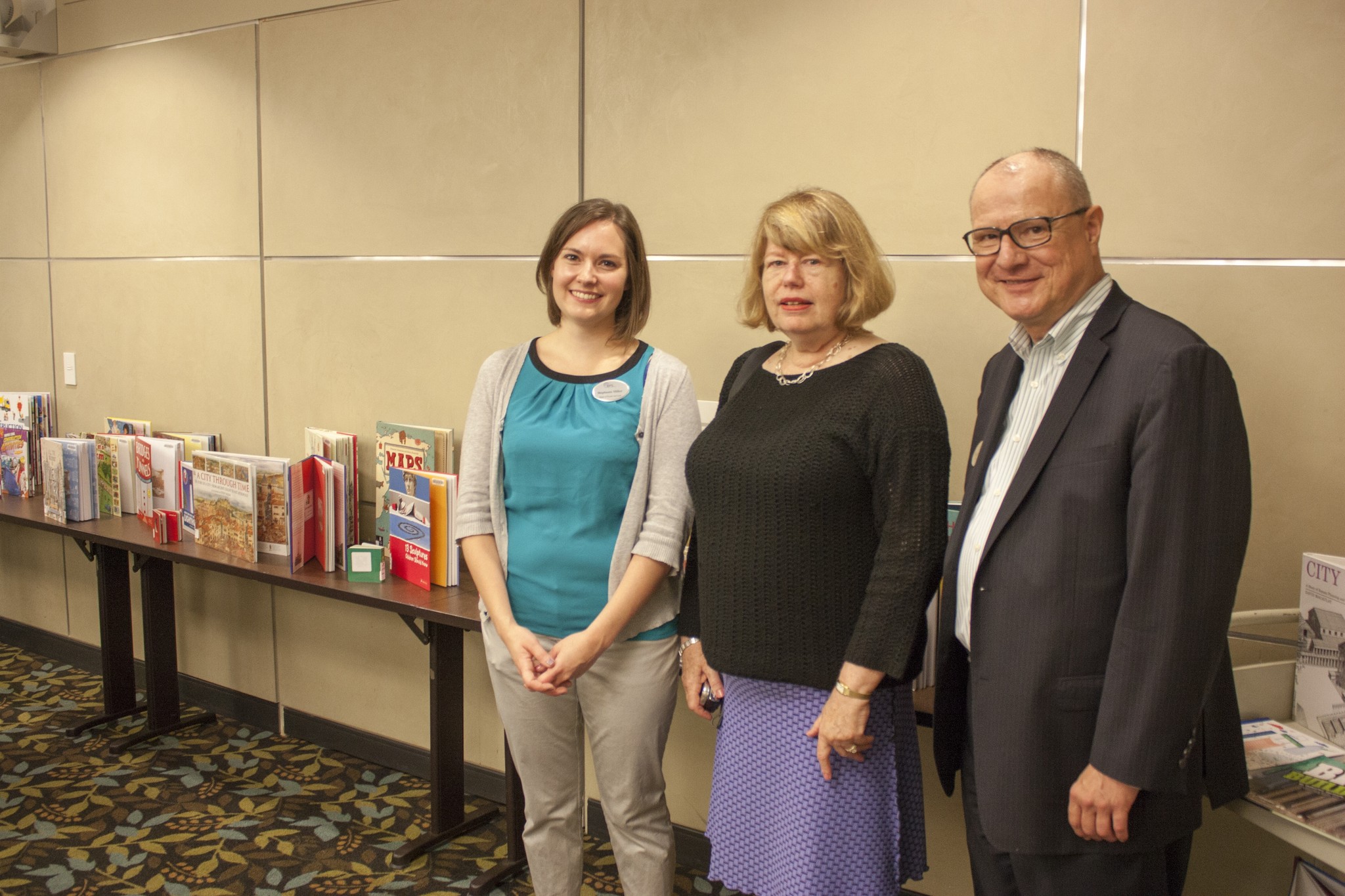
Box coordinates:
[774,328,852,387]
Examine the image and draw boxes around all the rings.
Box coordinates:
[846,745,858,755]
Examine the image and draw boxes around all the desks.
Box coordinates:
[0,488,558,895]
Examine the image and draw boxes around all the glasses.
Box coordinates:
[962,207,1088,256]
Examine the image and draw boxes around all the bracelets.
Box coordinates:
[677,638,701,656]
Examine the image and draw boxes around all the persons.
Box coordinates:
[453,198,705,896]
[676,186,948,896]
[931,151,1249,896]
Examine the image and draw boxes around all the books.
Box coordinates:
[0,389,462,592]
[1237,549,1345,896]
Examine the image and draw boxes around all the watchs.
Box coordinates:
[835,678,872,701]
